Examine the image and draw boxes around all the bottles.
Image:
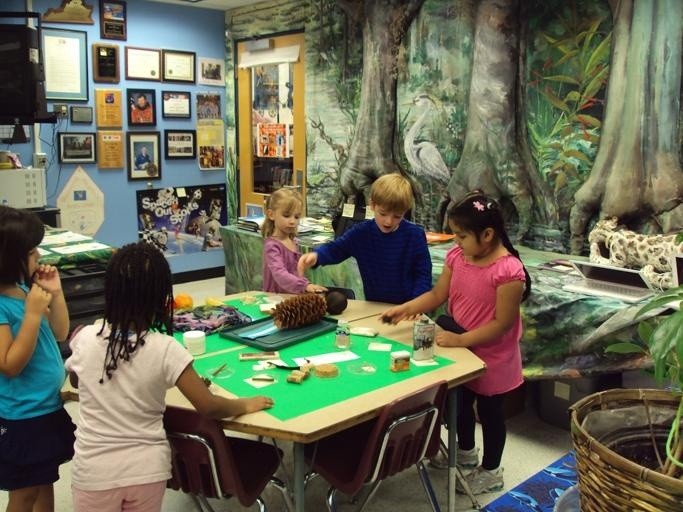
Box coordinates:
[410,318,434,361]
[336,320,349,349]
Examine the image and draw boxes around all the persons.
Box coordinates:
[203,62,221,80]
[83,137,92,149]
[198,95,223,121]
[73,138,81,149]
[0,204,76,511]
[378,188,531,496]
[200,145,224,169]
[202,226,220,251]
[132,93,151,123]
[259,186,328,293]
[173,223,195,254]
[136,144,152,172]
[164,92,173,102]
[296,173,433,303]
[153,226,168,250]
[68,244,275,512]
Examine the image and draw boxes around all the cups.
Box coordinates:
[182,330,206,356]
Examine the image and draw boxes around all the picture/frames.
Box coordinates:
[93,44,120,84]
[127,89,157,125]
[99,0,128,41]
[162,91,192,118]
[165,129,197,159]
[124,47,161,81]
[35,25,89,101]
[58,132,96,164]
[126,132,161,180]
[161,49,196,83]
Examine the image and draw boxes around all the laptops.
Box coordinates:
[561,257,655,304]
[671,255,682,287]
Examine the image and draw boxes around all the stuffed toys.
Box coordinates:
[588,215,683,291]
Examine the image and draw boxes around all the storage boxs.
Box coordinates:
[535,373,615,431]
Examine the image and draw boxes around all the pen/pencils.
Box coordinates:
[213,363,227,376]
[252,378,274,381]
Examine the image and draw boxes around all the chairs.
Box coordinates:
[299,382,448,512]
[162,406,291,512]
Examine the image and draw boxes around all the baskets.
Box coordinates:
[568,389,683,512]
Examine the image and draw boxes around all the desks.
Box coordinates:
[61,289,490,512]
[218,215,683,385]
[478,451,579,511]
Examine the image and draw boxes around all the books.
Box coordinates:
[295,217,335,236]
[237,217,268,233]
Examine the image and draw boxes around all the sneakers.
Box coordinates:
[456,465,503,495]
[430,442,480,469]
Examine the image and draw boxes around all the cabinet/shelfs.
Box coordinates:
[33,207,116,329]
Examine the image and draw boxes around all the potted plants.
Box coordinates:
[568,231,683,512]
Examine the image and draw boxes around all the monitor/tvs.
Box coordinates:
[0,11,57,125]
